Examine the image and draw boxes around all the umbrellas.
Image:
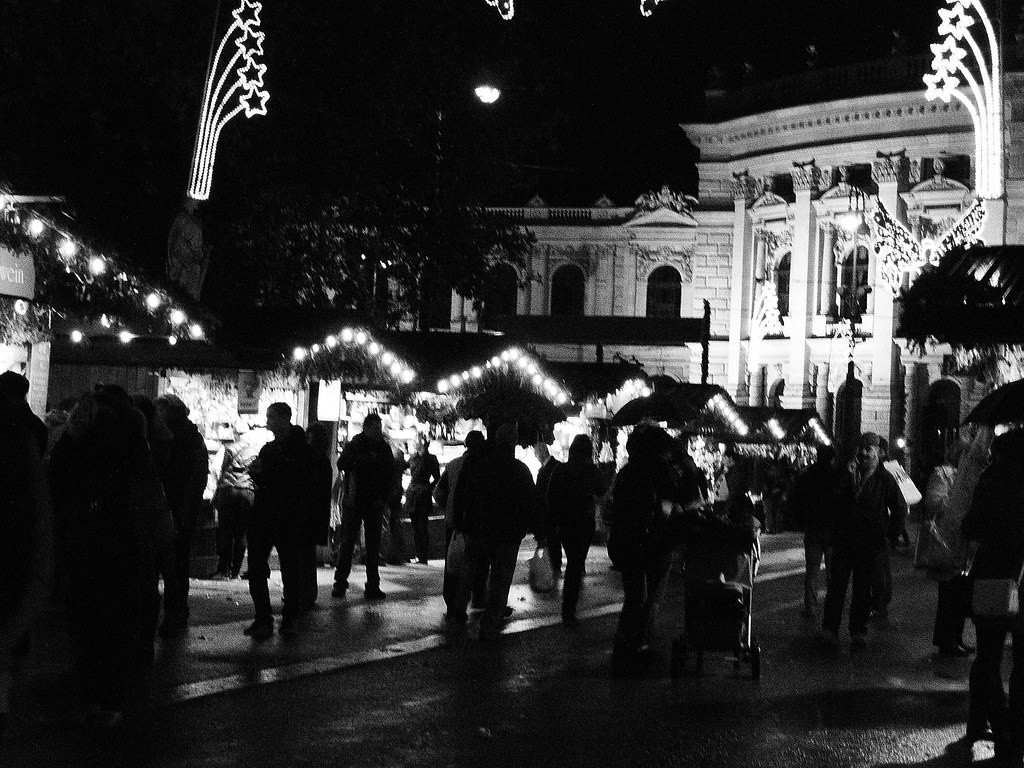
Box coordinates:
[962,378,1024,423]
[608,394,700,427]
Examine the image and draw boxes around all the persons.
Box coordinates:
[0,372,1024,750]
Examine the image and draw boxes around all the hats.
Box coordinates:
[856,433,880,445]
[571,436,592,457]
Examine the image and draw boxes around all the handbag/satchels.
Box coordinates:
[446,529,465,576]
[529,547,555,593]
[915,520,976,577]
[973,579,1018,616]
[218,472,254,507]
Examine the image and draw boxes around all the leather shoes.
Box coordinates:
[939,644,977,654]
[968,723,993,737]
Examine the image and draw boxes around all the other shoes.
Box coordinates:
[821,629,838,645]
[505,607,512,617]
[564,609,578,628]
[365,588,386,597]
[279,623,298,633]
[72,707,121,729]
[244,622,273,634]
[868,608,887,618]
[800,607,816,618]
[851,634,866,645]
[479,635,509,648]
[213,574,237,580]
[331,583,348,596]
